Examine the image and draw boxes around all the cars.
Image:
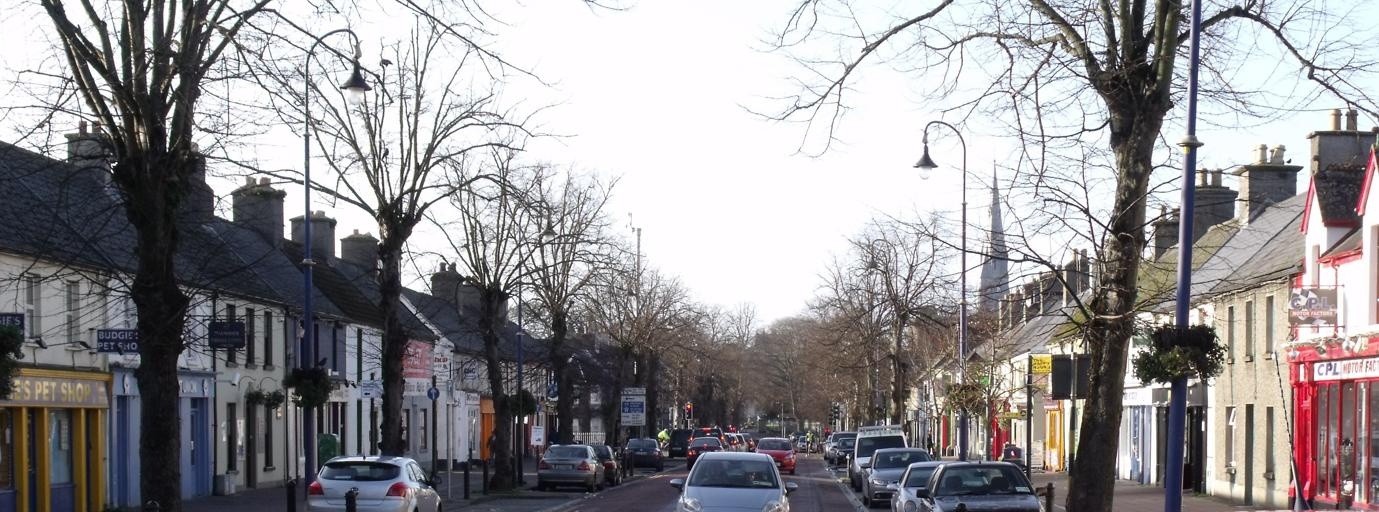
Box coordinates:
[536,426,824,511]
[819,424,1047,512]
[1317,432,1379,499]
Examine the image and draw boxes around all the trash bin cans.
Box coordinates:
[317,433,341,475]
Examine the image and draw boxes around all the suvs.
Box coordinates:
[306,454,444,511]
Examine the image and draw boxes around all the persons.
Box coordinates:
[790,431,798,451]
[657,426,672,448]
[926,433,934,456]
[805,428,816,455]
[486,430,496,465]
[703,461,729,485]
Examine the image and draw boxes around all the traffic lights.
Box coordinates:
[834,405,838,419]
[828,408,832,425]
[686,402,691,419]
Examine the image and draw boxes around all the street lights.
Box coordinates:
[915,114,970,458]
[608,268,636,414]
[512,197,559,486]
[303,28,373,481]
[868,236,901,310]
